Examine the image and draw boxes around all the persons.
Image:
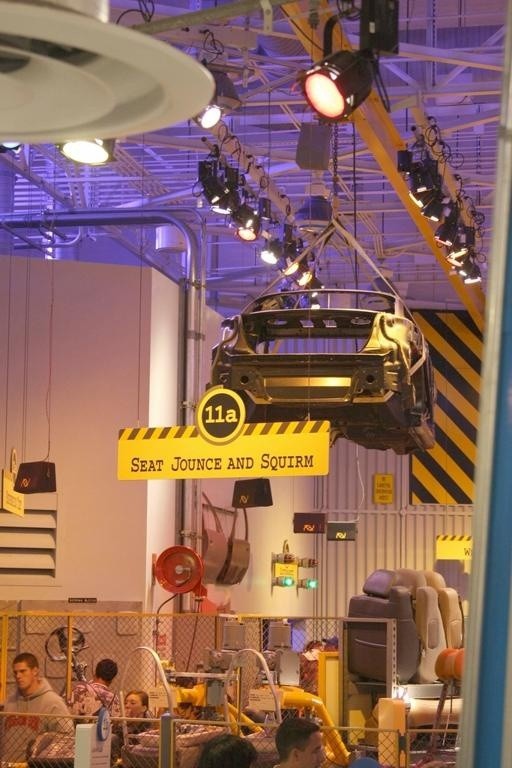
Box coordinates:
[111,689,155,767]
[194,733,259,767]
[322,636,339,651]
[273,718,326,768]
[171,676,199,706]
[1,652,75,768]
[63,658,122,729]
[297,640,322,696]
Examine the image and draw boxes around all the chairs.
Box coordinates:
[346,569,466,686]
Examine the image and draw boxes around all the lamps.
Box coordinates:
[193,104,222,131]
[397,148,482,286]
[271,552,320,596]
[198,160,325,297]
[301,49,380,122]
[59,133,116,167]
